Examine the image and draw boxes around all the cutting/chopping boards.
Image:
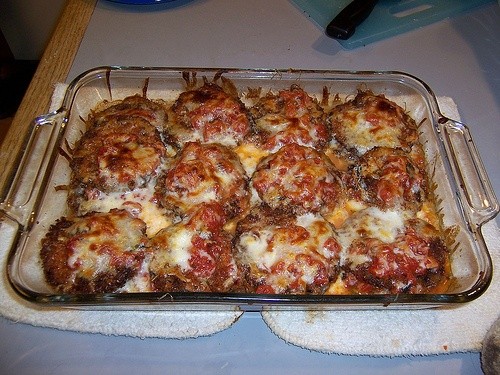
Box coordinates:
[288,0,497,49]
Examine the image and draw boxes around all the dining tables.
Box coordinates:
[0,0,500,375]
